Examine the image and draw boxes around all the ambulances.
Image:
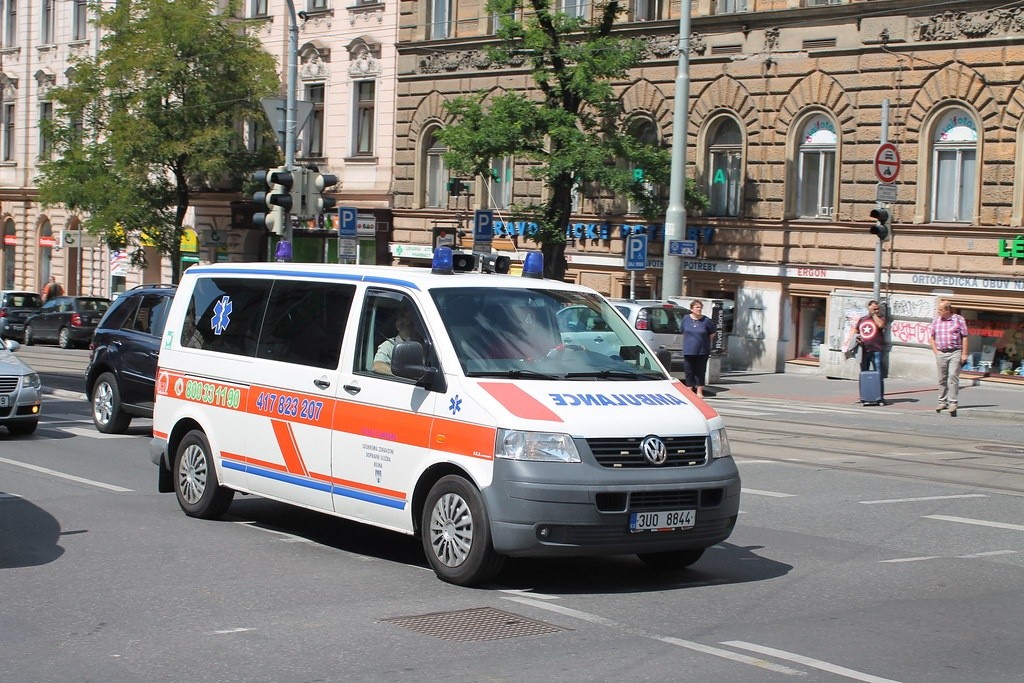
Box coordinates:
[148,241,741,590]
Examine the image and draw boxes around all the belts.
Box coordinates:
[937,349,960,353]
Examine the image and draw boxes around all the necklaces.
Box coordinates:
[692,317,698,327]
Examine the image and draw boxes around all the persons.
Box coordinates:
[44,276,64,303]
[372,305,429,380]
[680,300,715,398]
[930,301,969,413]
[486,304,551,360]
[854,301,886,406]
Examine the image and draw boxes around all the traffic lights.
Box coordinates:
[868,208,893,245]
[250,170,286,237]
[307,169,336,217]
[269,169,302,216]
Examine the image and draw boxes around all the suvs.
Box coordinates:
[84,282,179,434]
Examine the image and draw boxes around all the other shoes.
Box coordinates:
[936,403,947,410]
[881,398,890,405]
[949,404,958,412]
[697,392,704,398]
[854,398,860,405]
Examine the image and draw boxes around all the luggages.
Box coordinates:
[859,371,883,407]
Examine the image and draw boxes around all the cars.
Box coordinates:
[0,338,41,435]
[554,300,692,381]
[25,296,114,349]
[0,290,44,344]
